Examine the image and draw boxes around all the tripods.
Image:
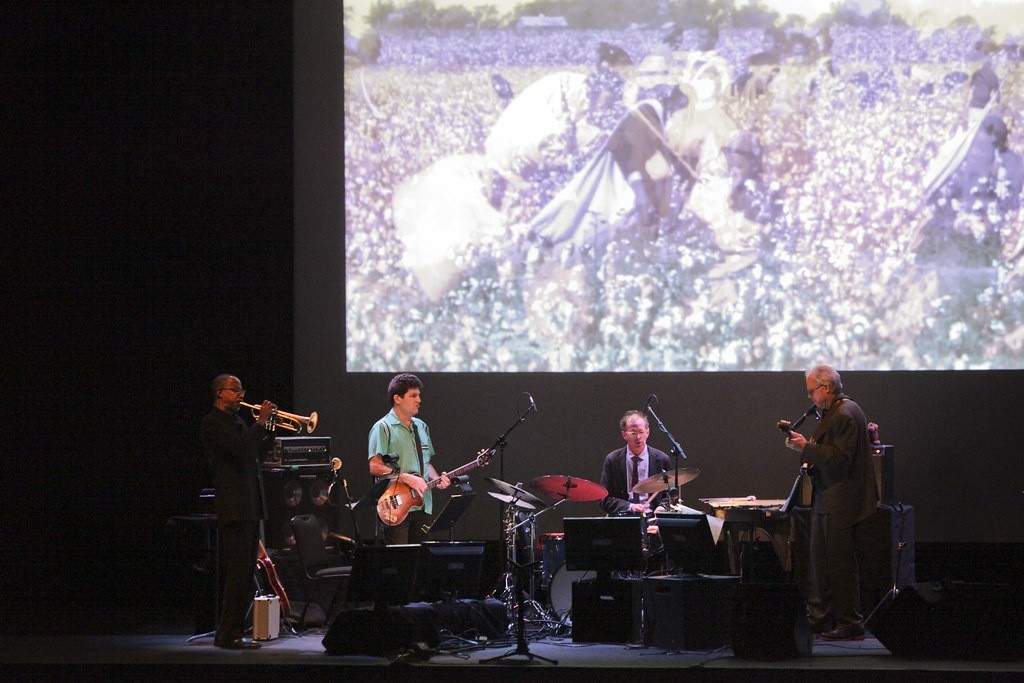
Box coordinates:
[478,486,571,664]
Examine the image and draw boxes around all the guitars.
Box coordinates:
[255,539,292,619]
[775,419,818,506]
[376,447,498,528]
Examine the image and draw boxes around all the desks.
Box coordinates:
[170,513,225,644]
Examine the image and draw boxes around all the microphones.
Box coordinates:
[527,392,537,412]
[326,458,341,494]
[642,394,653,414]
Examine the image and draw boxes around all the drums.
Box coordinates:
[708,499,795,576]
[549,560,643,626]
[538,531,567,591]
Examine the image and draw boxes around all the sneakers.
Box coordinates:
[805,613,833,633]
[821,627,865,641]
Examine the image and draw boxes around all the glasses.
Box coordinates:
[215,388,245,396]
[626,430,647,437]
[808,384,824,396]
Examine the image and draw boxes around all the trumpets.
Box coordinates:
[238,399,319,435]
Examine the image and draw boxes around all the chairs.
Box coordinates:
[290,514,355,634]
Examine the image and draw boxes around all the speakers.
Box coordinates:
[728,581,1024,662]
[320,606,441,658]
[871,445,895,507]
[261,466,338,555]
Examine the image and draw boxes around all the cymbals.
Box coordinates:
[632,467,702,494]
[483,476,550,509]
[529,473,609,503]
[486,491,537,510]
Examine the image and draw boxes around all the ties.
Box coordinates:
[631,456,642,502]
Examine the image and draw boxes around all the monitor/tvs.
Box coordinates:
[346,540,487,608]
[654,511,725,576]
[563,518,644,571]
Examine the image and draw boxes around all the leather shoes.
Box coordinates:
[214,637,262,649]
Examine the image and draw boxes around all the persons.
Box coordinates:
[959,117,1024,191]
[598,411,672,516]
[484,20,834,210]
[970,61,1001,109]
[368,374,450,545]
[785,364,877,641]
[200,374,278,651]
